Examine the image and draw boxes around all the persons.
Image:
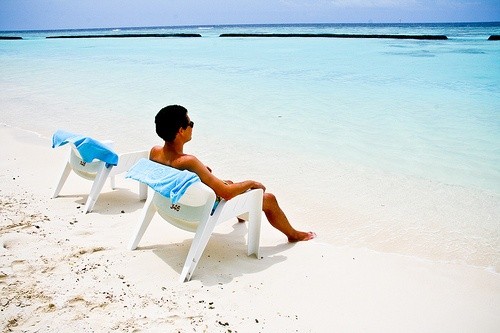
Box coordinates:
[149,105,317,244]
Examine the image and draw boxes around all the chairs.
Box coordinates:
[48,130,149,216]
[124,156,265,284]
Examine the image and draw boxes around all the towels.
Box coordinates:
[51,129,120,167]
[126,158,219,216]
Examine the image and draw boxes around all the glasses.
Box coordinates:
[186,120,193,127]
